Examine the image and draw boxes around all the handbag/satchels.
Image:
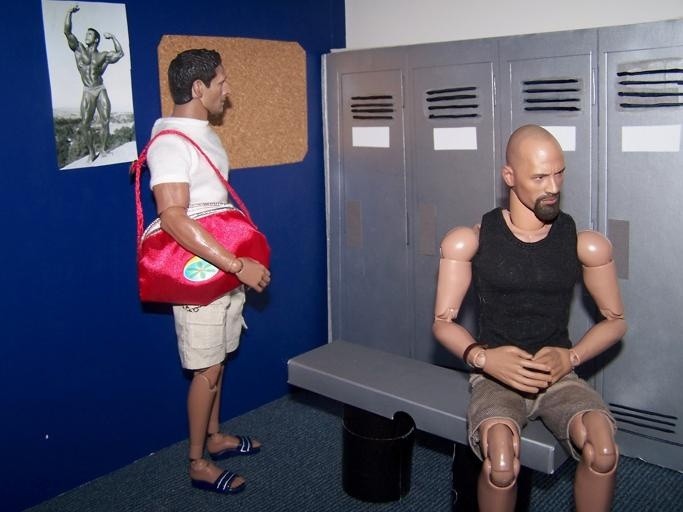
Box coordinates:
[134,200,277,308]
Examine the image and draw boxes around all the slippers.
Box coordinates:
[209,432,263,462]
[189,470,247,494]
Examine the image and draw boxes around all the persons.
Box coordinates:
[61,3,125,165]
[146,47,272,495]
[429,125,626,512]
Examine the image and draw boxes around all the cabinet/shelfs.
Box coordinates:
[320,38,501,378]
[500,21,683,476]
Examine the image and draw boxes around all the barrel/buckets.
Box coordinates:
[344,410,415,503]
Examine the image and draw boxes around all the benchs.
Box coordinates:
[287,338,571,512]
